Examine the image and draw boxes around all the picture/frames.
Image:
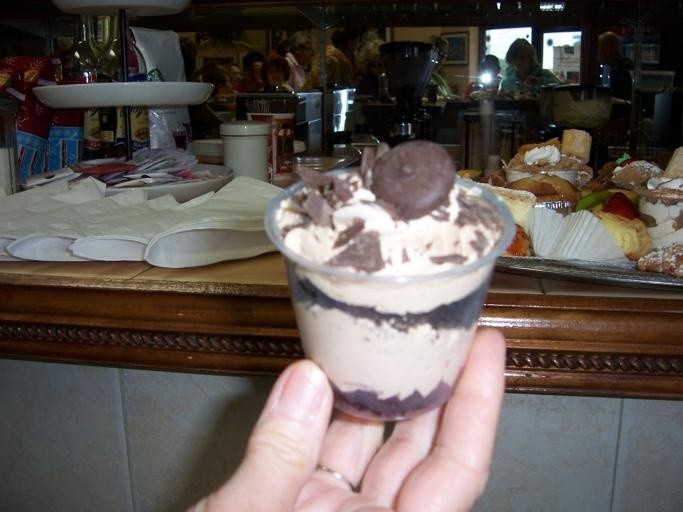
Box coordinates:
[439,31,470,67]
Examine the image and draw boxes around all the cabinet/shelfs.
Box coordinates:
[359,98,540,177]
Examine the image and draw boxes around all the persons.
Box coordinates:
[423,35,454,98]
[303,27,363,89]
[357,38,390,95]
[503,39,561,93]
[177,31,314,138]
[597,31,632,119]
[473,55,502,90]
[184,327,506,512]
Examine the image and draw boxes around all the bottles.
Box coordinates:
[0,98,23,196]
[69,14,150,161]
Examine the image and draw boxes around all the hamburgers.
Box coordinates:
[504,172,575,217]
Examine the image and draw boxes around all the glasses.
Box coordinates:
[304,47,314,52]
[368,62,382,68]
[438,50,449,60]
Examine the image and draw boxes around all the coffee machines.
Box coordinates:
[377,41,438,147]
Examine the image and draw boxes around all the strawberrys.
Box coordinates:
[602,193,637,220]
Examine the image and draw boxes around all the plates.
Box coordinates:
[192,139,223,164]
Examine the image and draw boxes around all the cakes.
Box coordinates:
[265,140,500,419]
[595,191,653,260]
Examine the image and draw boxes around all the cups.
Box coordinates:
[219,122,271,181]
[246,112,296,181]
[265,165,517,424]
[443,112,514,171]
